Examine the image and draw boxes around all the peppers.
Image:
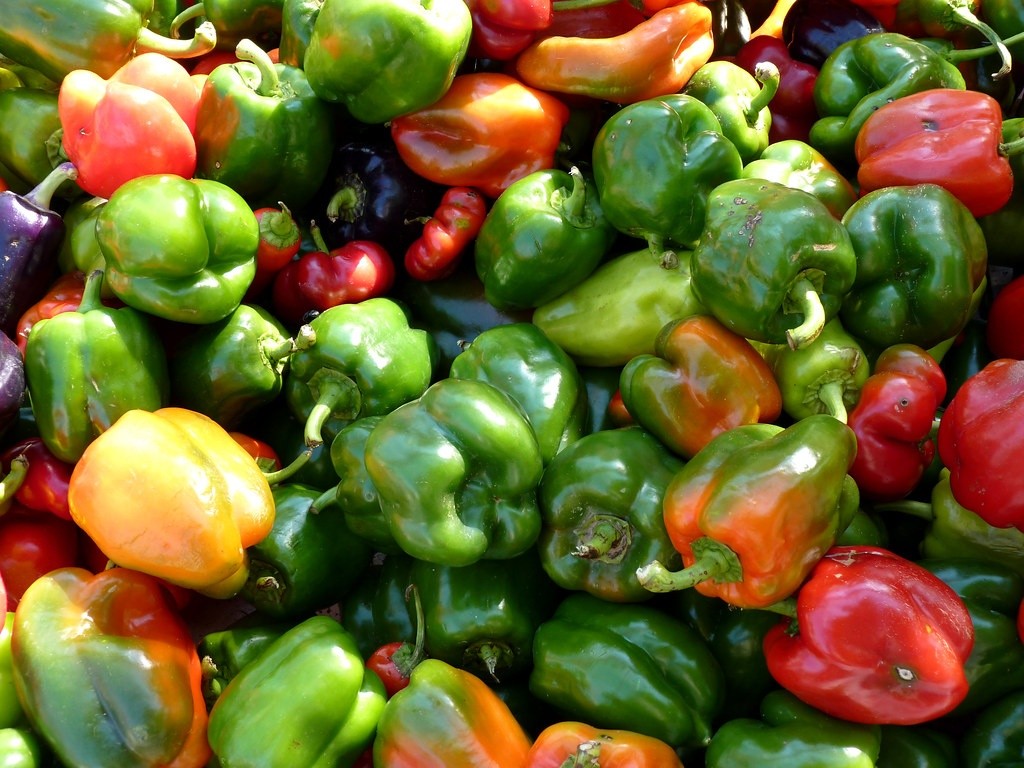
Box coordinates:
[0,0,1024,766]
